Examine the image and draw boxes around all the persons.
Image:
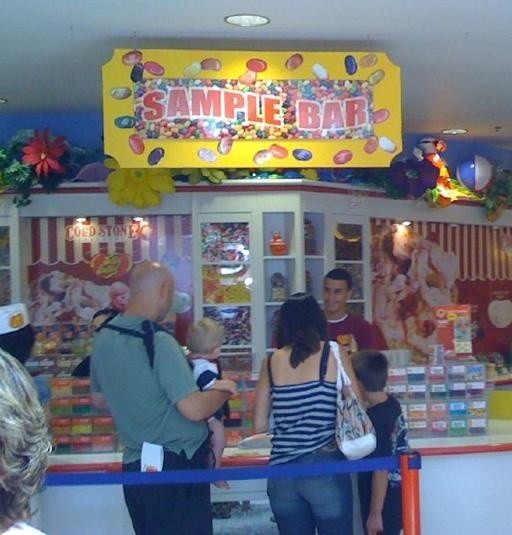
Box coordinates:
[253,292,365,535]
[92,281,129,330]
[319,268,377,362]
[187,316,238,489]
[349,350,409,535]
[89,261,233,535]
[0,348,54,535]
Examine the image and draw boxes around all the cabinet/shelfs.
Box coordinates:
[0,194,20,306]
[195,191,372,381]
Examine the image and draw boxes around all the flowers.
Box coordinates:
[0,129,71,207]
[105,158,175,208]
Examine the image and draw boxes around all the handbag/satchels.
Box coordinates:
[327,340,380,464]
